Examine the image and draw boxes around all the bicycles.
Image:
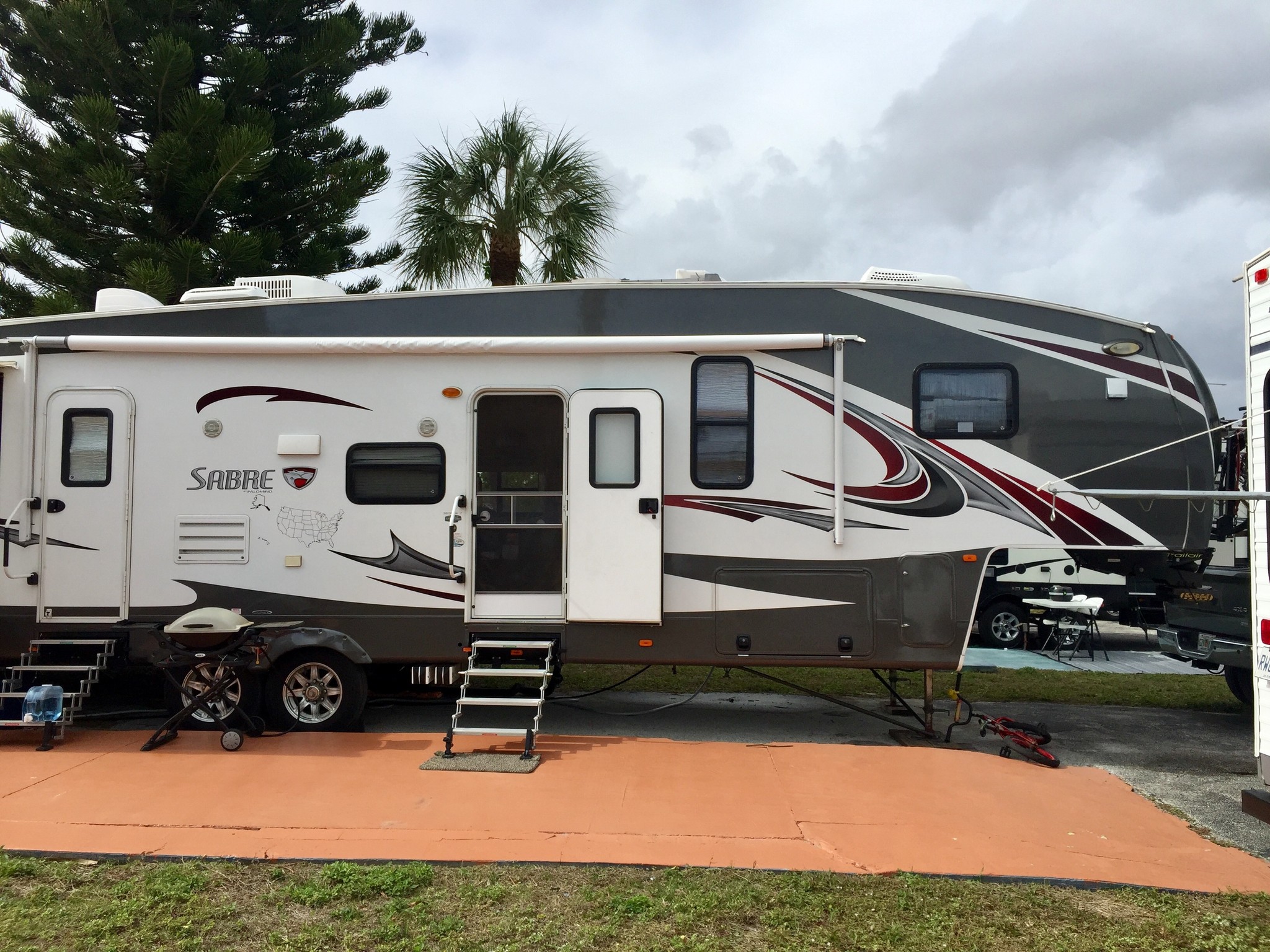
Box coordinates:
[944,673,1060,767]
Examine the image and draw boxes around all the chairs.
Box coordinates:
[1041,595,1111,660]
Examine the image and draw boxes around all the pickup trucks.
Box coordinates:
[1156,563,1254,705]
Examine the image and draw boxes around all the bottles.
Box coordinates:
[22,684,63,722]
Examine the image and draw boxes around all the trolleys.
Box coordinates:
[109,607,305,751]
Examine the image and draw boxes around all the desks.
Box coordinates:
[1024,599,1097,659]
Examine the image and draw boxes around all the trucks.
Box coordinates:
[972,437,1250,650]
